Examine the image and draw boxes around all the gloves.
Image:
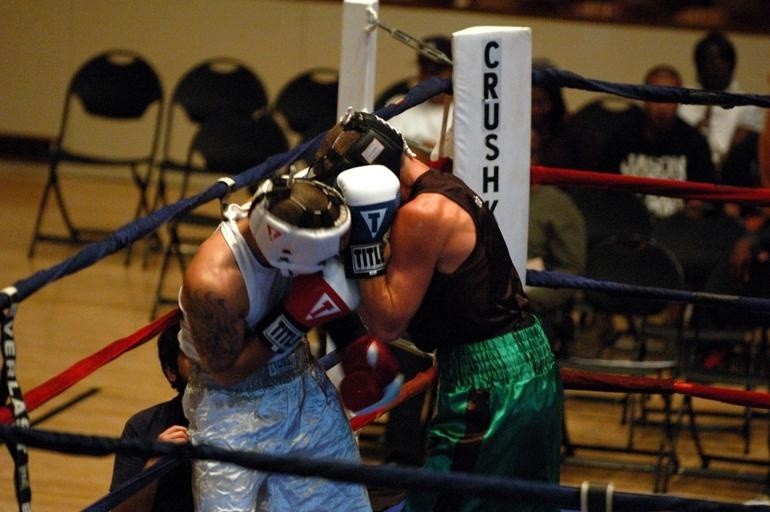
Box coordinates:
[339,333,406,417]
[254,258,362,354]
[331,165,402,279]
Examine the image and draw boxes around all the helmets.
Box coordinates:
[247,174,352,278]
[305,110,402,180]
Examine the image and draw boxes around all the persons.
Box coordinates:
[174,162,390,511]
[310,107,566,511]
[376,25,767,373]
[110,310,192,510]
